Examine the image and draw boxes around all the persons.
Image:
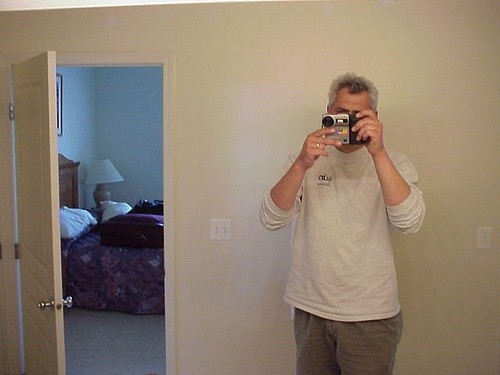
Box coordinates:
[258,73,426,375]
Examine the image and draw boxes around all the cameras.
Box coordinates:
[321,114,371,144]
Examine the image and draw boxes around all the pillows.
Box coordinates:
[60,206,97,239]
[101,201,132,225]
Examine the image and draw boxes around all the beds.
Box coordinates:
[59,154,163,315]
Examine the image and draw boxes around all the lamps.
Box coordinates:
[84,160,124,208]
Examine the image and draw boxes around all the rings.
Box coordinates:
[316,142,320,149]
